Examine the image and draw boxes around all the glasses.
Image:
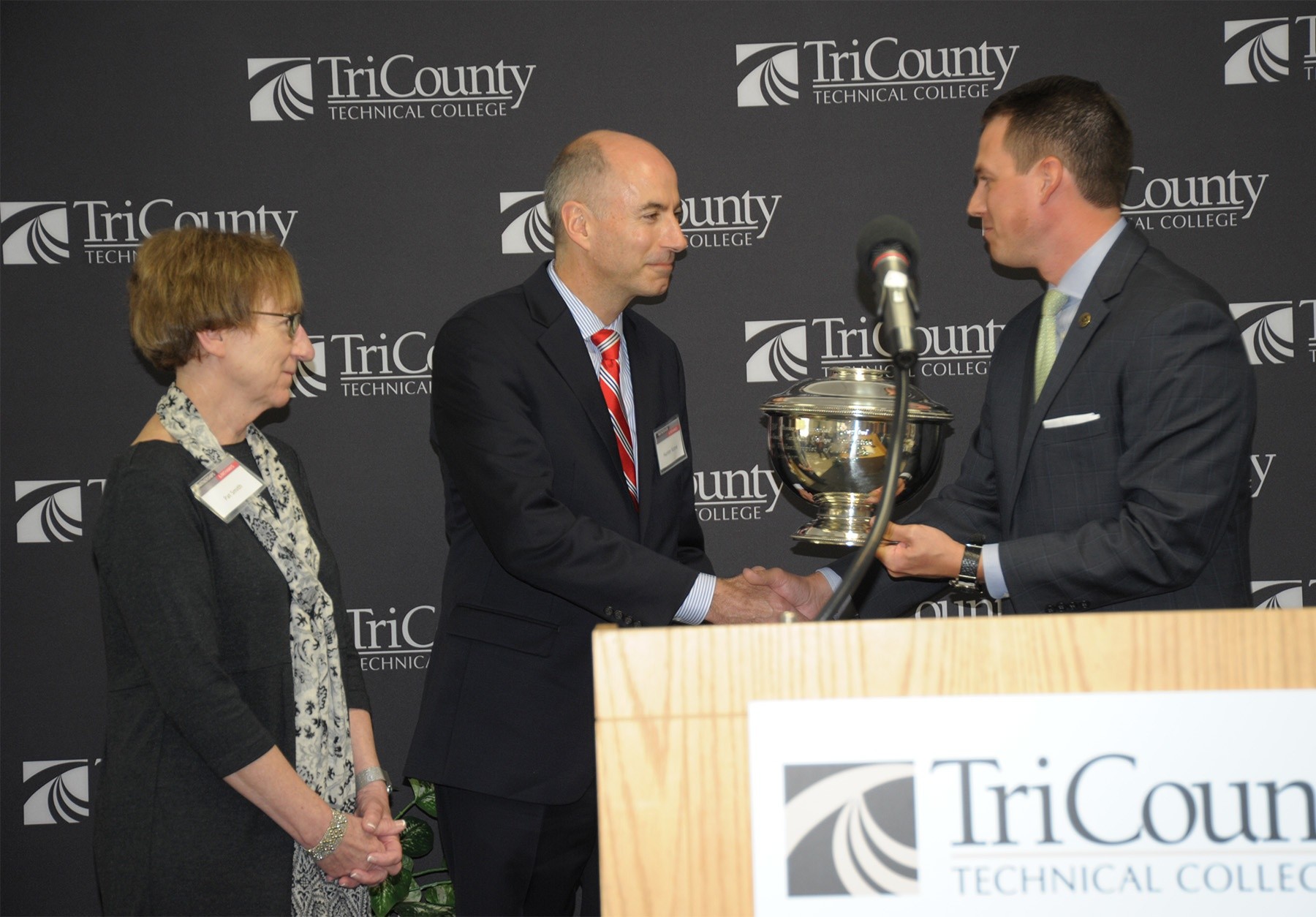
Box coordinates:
[248,310,305,334]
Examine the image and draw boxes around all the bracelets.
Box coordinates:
[356,767,393,796]
[302,810,348,861]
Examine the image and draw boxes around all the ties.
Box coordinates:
[591,329,640,511]
[1031,287,1070,403]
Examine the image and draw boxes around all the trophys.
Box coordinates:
[758,363,954,549]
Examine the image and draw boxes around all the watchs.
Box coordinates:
[947,543,985,595]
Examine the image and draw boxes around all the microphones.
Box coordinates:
[854,213,920,369]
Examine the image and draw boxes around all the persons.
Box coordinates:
[401,129,811,916]
[737,71,1253,616]
[765,414,947,525]
[93,225,410,916]
